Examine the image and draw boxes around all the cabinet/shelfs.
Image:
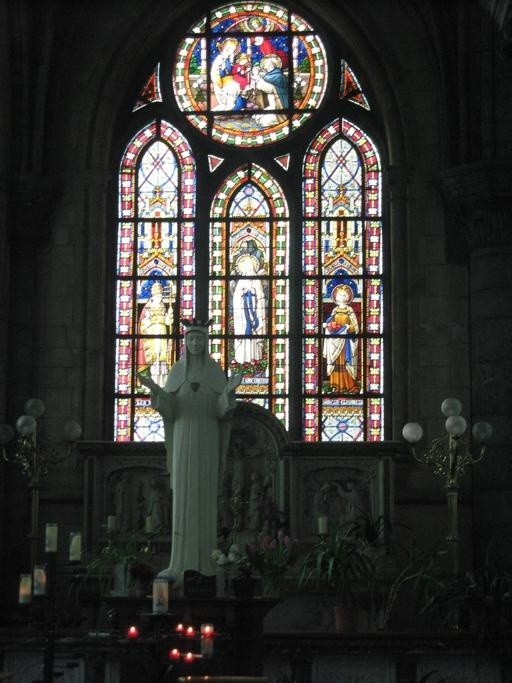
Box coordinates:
[99,592,282,681]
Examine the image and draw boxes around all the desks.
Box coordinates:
[42,629,234,682]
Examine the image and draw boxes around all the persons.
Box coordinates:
[134,318,245,579]
[210,17,289,127]
[322,283,361,398]
[137,280,173,376]
[120,461,375,545]
[230,259,265,365]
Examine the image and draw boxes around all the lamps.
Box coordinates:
[1,392,85,619]
[399,393,492,626]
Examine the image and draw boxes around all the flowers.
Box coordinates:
[247,530,292,562]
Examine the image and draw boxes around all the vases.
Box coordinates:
[258,560,282,598]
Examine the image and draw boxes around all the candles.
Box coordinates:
[12,513,173,613]
[318,516,328,534]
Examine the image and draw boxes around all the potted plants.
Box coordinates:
[293,517,387,632]
